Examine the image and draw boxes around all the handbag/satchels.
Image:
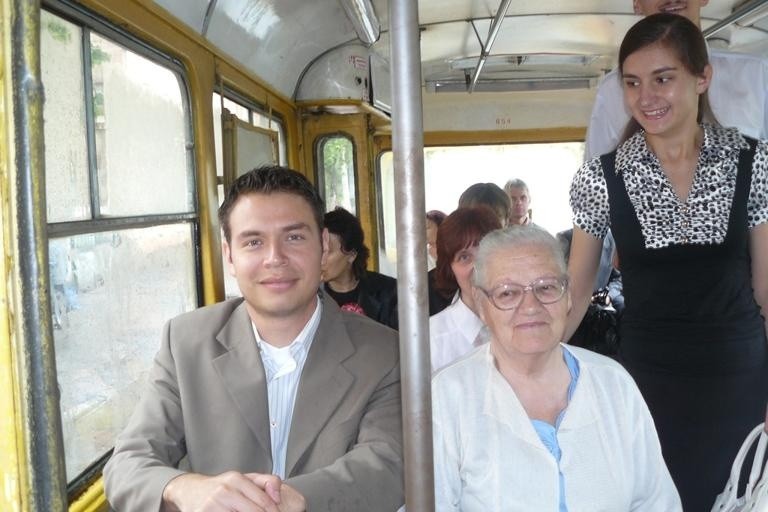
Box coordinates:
[713,426,768,511]
[583,288,615,358]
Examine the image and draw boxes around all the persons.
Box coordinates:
[459,183,511,228]
[504,180,538,227]
[561,12,768,512]
[584,0,767,270]
[429,224,683,512]
[426,210,448,271]
[318,207,400,328]
[556,225,625,361]
[104,166,406,510]
[428,207,504,374]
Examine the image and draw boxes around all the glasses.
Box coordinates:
[477,275,570,312]
